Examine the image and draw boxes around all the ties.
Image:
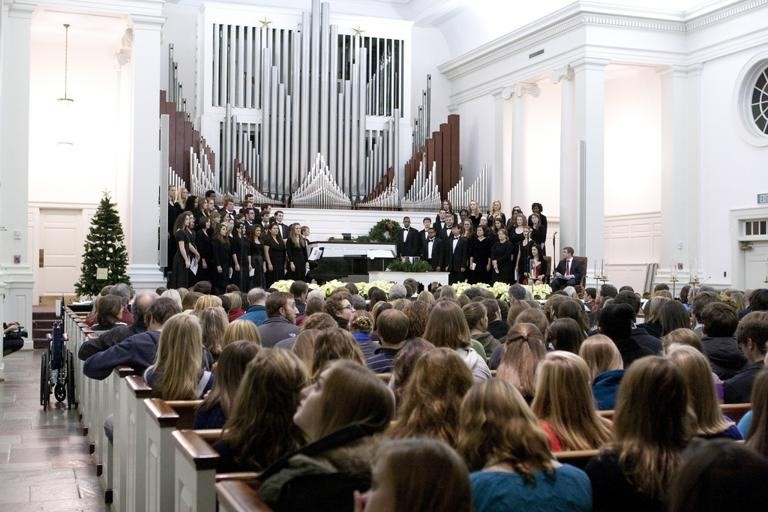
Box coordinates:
[566,260,569,274]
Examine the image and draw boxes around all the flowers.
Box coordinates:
[270,278,555,301]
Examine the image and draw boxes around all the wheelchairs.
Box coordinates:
[39,321,75,410]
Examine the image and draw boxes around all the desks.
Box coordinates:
[309,232,398,273]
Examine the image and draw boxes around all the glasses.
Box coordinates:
[337,303,352,310]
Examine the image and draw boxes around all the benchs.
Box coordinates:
[59,300,766,509]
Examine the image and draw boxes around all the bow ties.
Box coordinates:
[453,238,457,239]
[445,227,451,229]
[403,229,407,231]
[428,240,432,241]
[440,221,444,222]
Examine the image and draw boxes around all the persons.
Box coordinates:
[3,322,23,359]
[167,186,311,294]
[78,278,766,511]
[395,199,584,294]
